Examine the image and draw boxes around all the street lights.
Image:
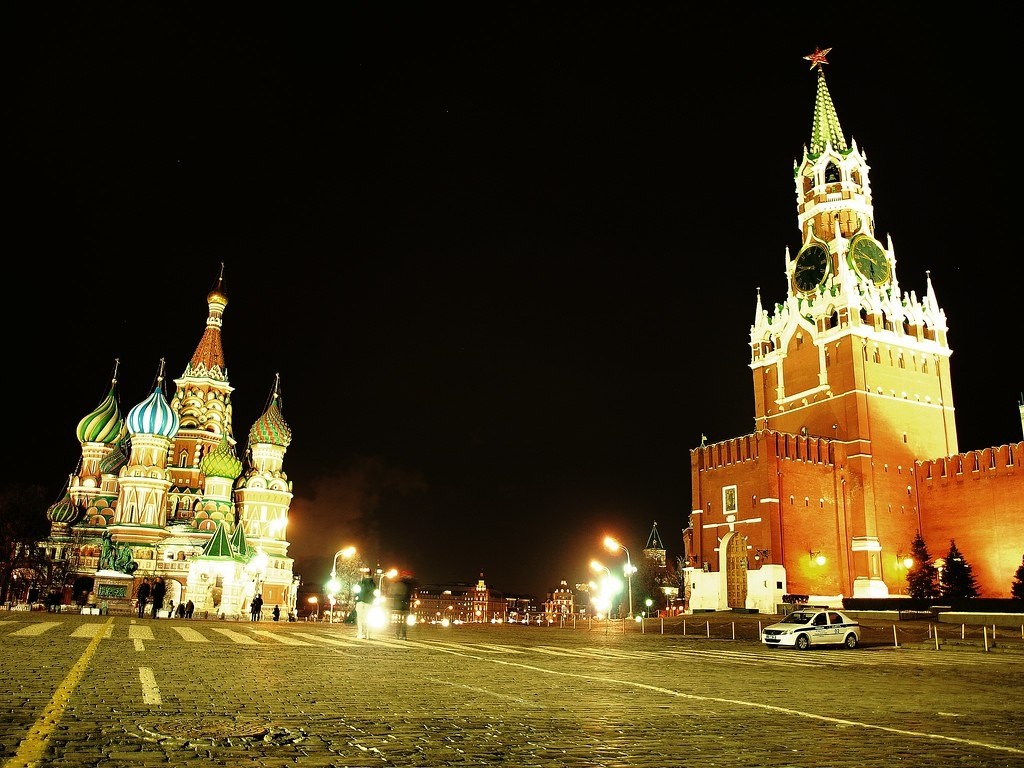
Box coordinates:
[605,539,633,620]
[378,569,397,606]
[444,605,453,623]
[592,562,611,620]
[330,548,356,623]
[664,588,671,616]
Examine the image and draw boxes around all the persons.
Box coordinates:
[44,577,295,622]
[395,621,408,641]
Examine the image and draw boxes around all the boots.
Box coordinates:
[137,605,141,618]
[152,608,156,619]
[141,605,144,618]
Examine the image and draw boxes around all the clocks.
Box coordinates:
[851,234,890,287]
[792,242,831,294]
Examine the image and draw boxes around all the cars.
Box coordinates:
[762,605,861,650]
